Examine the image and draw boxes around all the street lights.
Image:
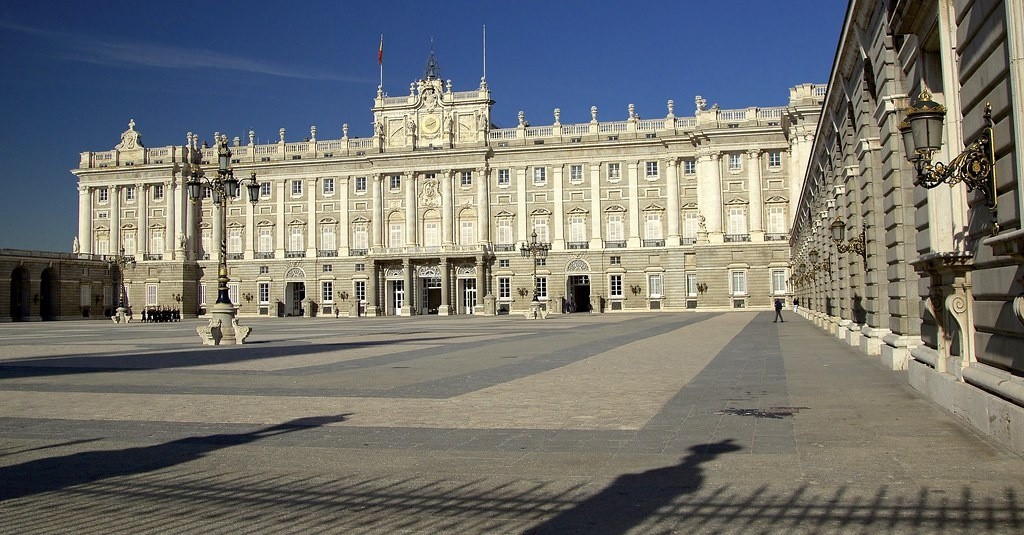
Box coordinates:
[185,133,259,346]
[520,230,549,320]
[108,244,137,325]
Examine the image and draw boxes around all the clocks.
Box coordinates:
[421,114,440,133]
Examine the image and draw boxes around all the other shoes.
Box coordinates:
[773,321,777,322]
[781,321,783,322]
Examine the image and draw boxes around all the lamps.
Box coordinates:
[899,85,999,235]
[518,287,528,298]
[630,285,641,296]
[96,295,104,305]
[241,293,253,303]
[33,294,39,305]
[696,283,707,294]
[172,293,183,304]
[786,249,831,289]
[828,215,868,272]
[337,291,348,301]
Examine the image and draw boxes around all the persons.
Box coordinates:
[587,304,593,314]
[565,301,571,314]
[793,298,798,313]
[140,308,180,323]
[335,306,340,318]
[772,298,784,323]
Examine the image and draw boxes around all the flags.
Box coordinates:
[377,35,383,64]
